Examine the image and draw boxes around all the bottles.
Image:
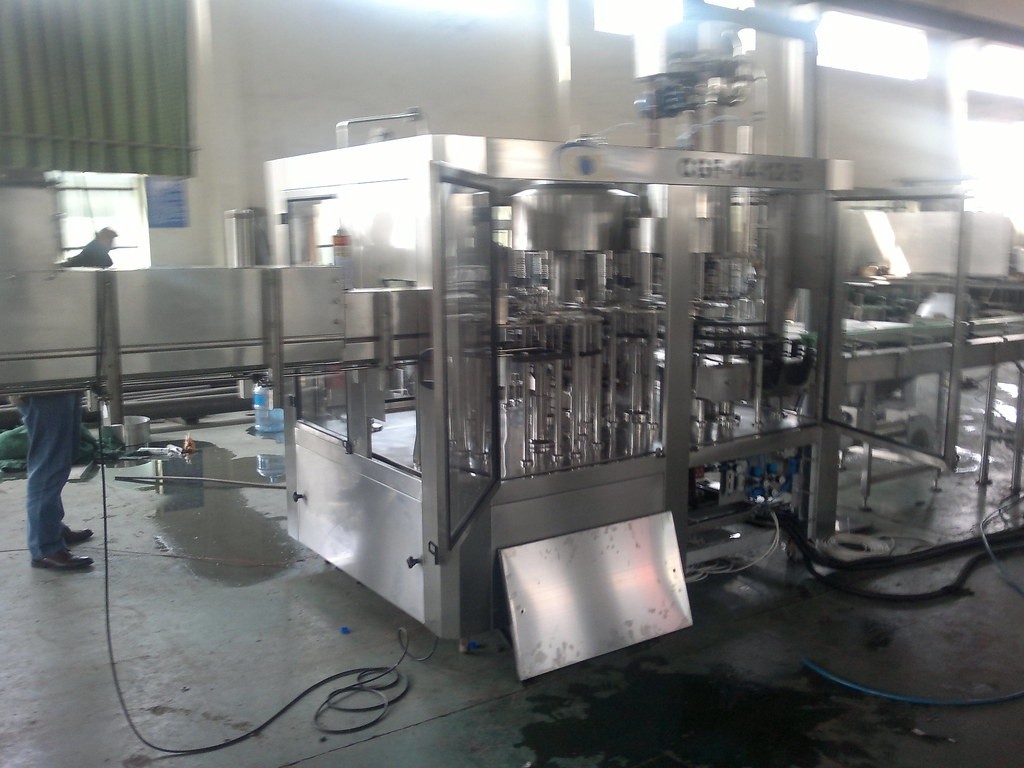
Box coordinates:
[250,371,284,432]
[255,455,285,484]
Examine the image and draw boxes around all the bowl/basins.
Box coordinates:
[124,416,150,446]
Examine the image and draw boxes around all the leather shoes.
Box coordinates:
[61,526,94,544]
[31,548,94,569]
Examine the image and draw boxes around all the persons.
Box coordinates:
[0,170,119,571]
